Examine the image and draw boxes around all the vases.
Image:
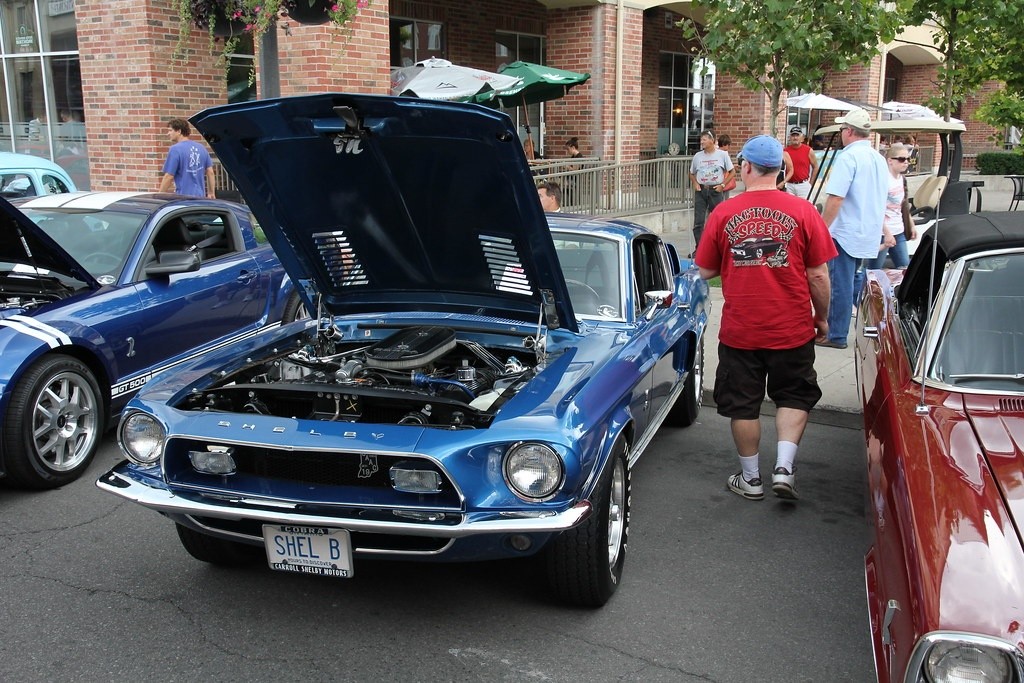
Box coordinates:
[281,0,337,25]
[198,4,255,36]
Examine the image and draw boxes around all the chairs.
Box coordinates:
[557,249,617,307]
[943,328,1024,382]
[1004,176,1024,211]
[151,217,194,263]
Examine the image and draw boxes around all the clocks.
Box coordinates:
[668,143,680,156]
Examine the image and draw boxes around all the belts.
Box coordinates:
[790,179,808,184]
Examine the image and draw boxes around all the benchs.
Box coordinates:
[189,226,232,260]
[908,175,948,225]
[948,295,1024,329]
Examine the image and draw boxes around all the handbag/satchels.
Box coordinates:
[902,175,912,240]
[723,171,736,191]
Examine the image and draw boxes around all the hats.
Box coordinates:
[790,127,802,135]
[737,135,783,167]
[834,108,871,130]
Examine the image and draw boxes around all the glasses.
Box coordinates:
[840,127,848,132]
[706,130,714,138]
[737,157,743,166]
[891,156,911,162]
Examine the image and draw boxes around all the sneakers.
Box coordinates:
[772,464,798,499]
[728,470,764,500]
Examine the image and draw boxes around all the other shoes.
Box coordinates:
[815,337,848,349]
[688,251,697,258]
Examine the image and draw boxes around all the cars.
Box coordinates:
[91,90,712,607]
[850,212,1023,683]
[0,151,109,274]
[0,188,313,492]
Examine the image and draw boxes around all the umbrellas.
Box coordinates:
[787,91,892,121]
[879,100,966,124]
[390,56,524,110]
[460,56,591,158]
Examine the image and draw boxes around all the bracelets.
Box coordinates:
[721,183,725,188]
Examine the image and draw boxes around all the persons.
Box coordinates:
[55,107,87,160]
[814,107,887,349]
[688,130,736,259]
[523,139,548,183]
[716,135,738,203]
[879,135,920,173]
[695,134,839,500]
[159,119,216,224]
[558,137,586,206]
[25,113,50,156]
[854,143,917,313]
[812,125,828,150]
[775,150,794,193]
[784,127,819,203]
[536,182,579,250]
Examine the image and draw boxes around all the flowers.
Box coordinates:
[169,0,372,98]
